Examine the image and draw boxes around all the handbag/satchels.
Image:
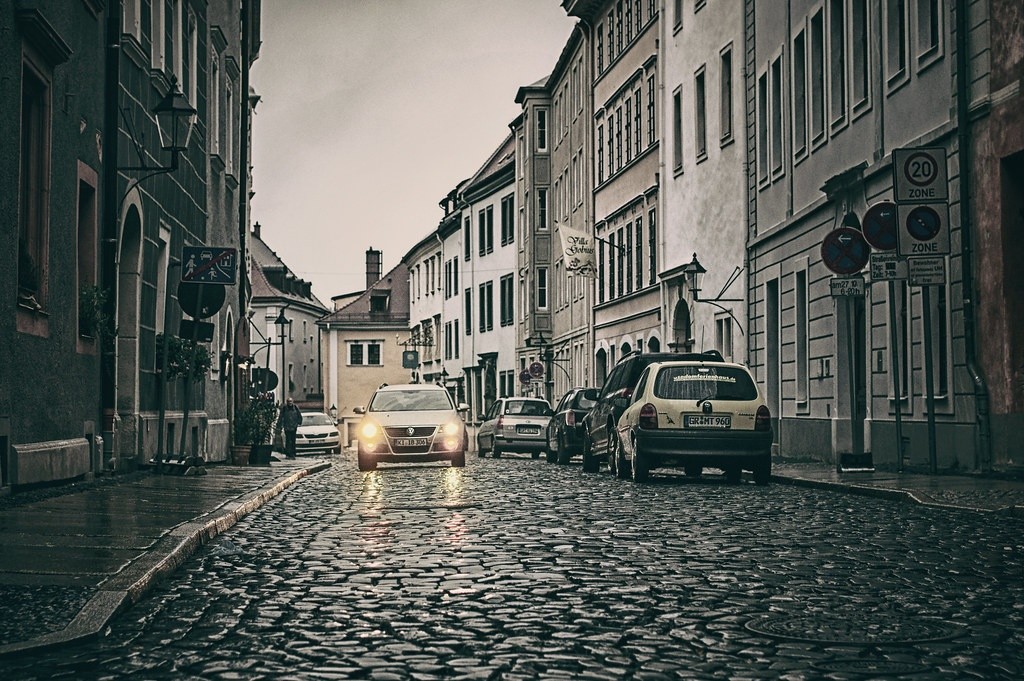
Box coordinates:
[275,434,282,452]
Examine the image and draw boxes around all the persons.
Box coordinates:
[276,398,302,459]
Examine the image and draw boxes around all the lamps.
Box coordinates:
[119,74,198,207]
[683,251,745,337]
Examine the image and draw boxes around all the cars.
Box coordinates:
[354,383,469,470]
[477,396,554,457]
[614,358,774,485]
[275,411,342,455]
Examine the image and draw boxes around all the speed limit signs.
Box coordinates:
[905,153,941,187]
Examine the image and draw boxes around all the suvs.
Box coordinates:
[543,386,603,465]
[580,349,726,477]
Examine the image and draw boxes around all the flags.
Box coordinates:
[556,222,598,279]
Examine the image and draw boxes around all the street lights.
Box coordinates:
[274,310,289,405]
